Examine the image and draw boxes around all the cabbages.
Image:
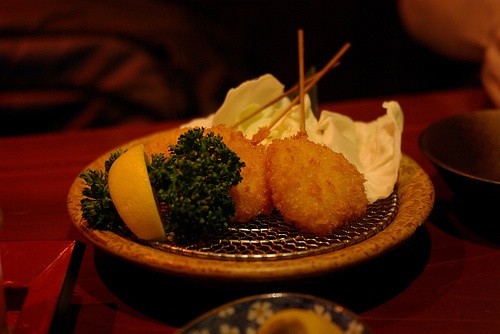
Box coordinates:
[190,73,404,205]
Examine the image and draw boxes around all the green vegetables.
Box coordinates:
[78,127,247,244]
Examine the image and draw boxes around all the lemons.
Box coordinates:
[108,144,167,243]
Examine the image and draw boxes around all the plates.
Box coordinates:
[66,127,434,277]
[179,291,365,334]
[0,239,77,334]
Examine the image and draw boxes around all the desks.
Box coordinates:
[0,84,500,334]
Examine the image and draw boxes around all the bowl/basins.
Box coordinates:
[417,109,500,207]
